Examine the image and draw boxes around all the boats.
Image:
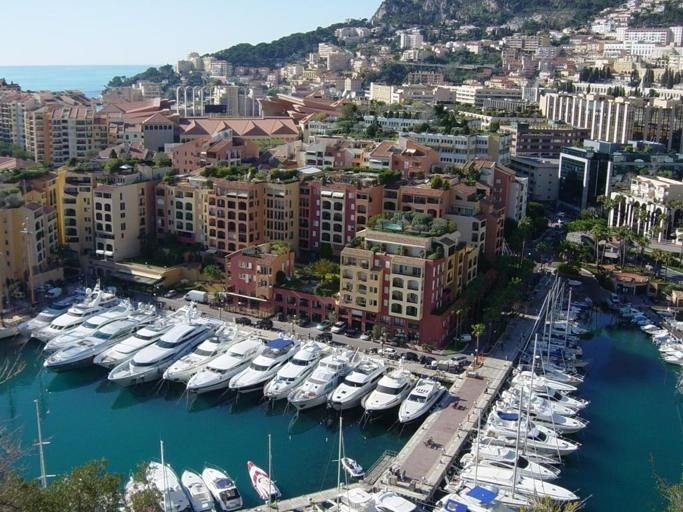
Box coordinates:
[263,340,330,400]
[621,307,683,365]
[434,427,578,510]
[263,342,321,401]
[246,460,281,503]
[619,305,683,365]
[363,356,418,414]
[361,366,419,412]
[483,293,593,429]
[432,292,595,512]
[247,460,280,498]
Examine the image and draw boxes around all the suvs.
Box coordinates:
[378,348,397,357]
[254,318,273,330]
[315,333,332,342]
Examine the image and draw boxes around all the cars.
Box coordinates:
[365,348,377,354]
[331,321,346,334]
[164,290,176,298]
[360,331,372,341]
[316,320,331,331]
[401,352,418,360]
[235,317,251,325]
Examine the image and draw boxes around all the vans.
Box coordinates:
[450,356,469,367]
[183,290,208,304]
[45,288,62,300]
[453,334,471,344]
[610,293,618,303]
